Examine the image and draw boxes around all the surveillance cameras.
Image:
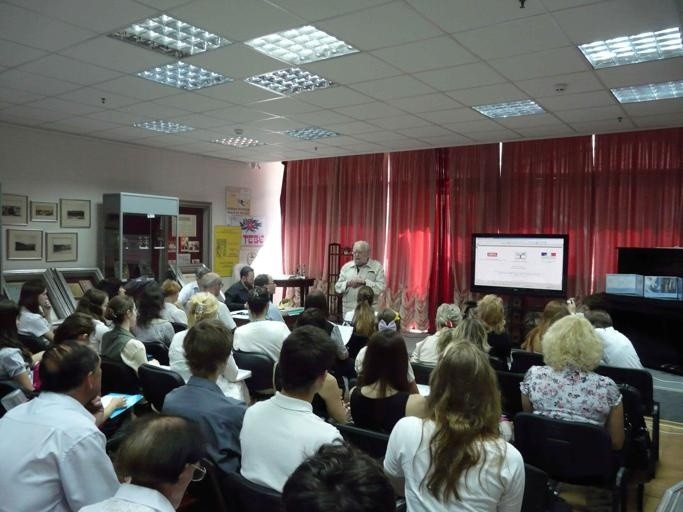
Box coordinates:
[100,97,107,105]
[618,116,622,123]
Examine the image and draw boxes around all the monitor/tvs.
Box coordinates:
[469,233,569,299]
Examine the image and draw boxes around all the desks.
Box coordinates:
[272,278,318,308]
[230,305,304,329]
[585,247,682,375]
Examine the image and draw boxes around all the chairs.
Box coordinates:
[496,370,526,417]
[511,349,543,368]
[139,363,185,409]
[171,322,188,332]
[203,451,282,512]
[618,383,657,479]
[336,423,390,461]
[101,361,139,397]
[598,364,661,456]
[524,463,564,510]
[513,412,644,510]
[234,349,274,390]
[489,357,509,369]
[144,340,168,363]
[19,335,47,350]
[410,362,435,385]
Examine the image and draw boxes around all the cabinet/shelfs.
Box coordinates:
[102,191,179,283]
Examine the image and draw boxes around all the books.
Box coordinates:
[280,307,306,316]
[231,309,251,320]
[69,278,96,306]
[235,368,253,383]
[100,391,144,419]
[327,319,355,346]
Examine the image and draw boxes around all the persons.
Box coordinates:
[235,287,294,362]
[356,308,421,394]
[242,326,346,493]
[346,286,379,338]
[280,444,396,511]
[0,299,37,392]
[385,342,526,512]
[76,289,111,352]
[168,292,252,402]
[168,323,246,473]
[0,342,121,512]
[351,331,431,435]
[526,300,571,353]
[461,301,478,319]
[160,279,190,327]
[477,295,514,368]
[200,272,238,332]
[17,280,56,344]
[99,296,160,378]
[306,290,357,381]
[522,316,645,511]
[569,299,645,369]
[271,308,349,422]
[97,275,128,299]
[454,321,491,358]
[80,416,204,512]
[226,267,255,309]
[254,275,288,325]
[34,314,97,395]
[336,241,387,320]
[411,303,463,369]
[134,280,175,346]
[178,268,227,306]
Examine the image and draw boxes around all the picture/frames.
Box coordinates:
[60,198,91,228]
[7,229,43,259]
[0,193,29,226]
[30,200,58,222]
[45,232,77,262]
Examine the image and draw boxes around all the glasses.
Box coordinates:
[266,281,275,286]
[352,251,364,256]
[191,464,207,482]
